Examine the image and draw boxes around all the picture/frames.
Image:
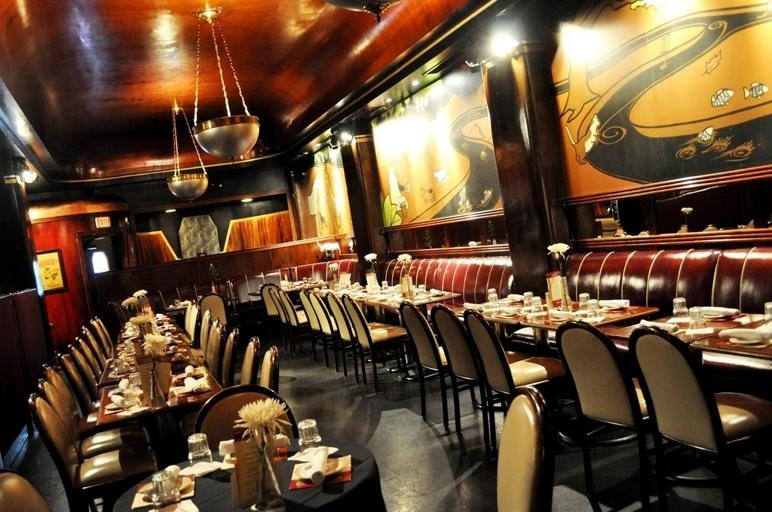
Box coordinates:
[34,248,68,296]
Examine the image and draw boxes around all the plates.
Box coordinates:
[728,336,760,344]
[106,309,208,411]
[294,458,344,480]
[703,313,722,320]
[143,475,192,491]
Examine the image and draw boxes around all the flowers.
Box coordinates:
[680,205,695,228]
[397,250,416,303]
[546,241,572,313]
[364,253,379,294]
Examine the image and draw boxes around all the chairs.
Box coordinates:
[0,271,772,512]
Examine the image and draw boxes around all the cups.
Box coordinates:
[297,418,321,452]
[688,306,706,332]
[187,432,212,468]
[312,281,427,301]
[487,290,602,322]
[672,297,689,322]
[152,469,176,503]
[765,301,771,322]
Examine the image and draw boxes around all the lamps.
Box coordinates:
[192,3,260,157]
[166,97,209,200]
